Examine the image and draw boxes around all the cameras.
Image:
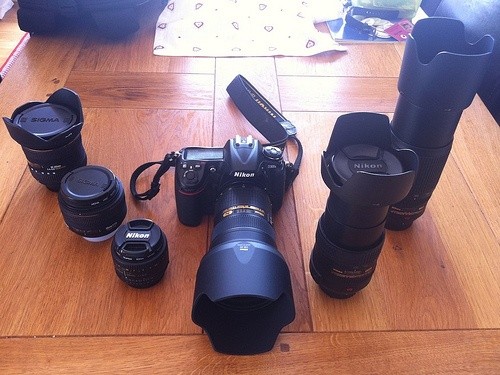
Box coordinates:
[176,135,295,356]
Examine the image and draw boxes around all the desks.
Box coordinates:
[0,0,500,374]
